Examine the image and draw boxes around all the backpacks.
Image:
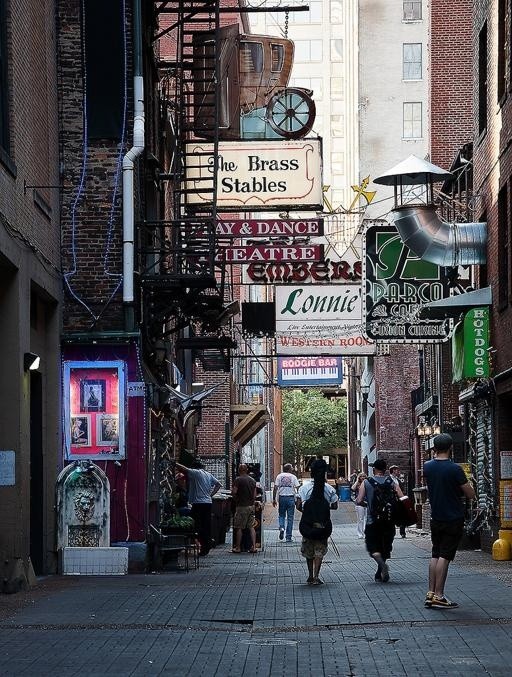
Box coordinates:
[298,458,332,540]
[366,476,399,524]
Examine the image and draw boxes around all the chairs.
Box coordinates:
[148,523,200,575]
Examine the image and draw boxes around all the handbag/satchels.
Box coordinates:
[395,496,419,527]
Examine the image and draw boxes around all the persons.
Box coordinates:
[311,453,330,483]
[355,458,405,582]
[174,471,201,547]
[422,431,477,609]
[388,463,406,539]
[395,465,404,483]
[272,462,300,542]
[228,463,258,552]
[168,456,223,555]
[85,385,100,408]
[72,417,88,446]
[349,471,369,539]
[295,463,338,585]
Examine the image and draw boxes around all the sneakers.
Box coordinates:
[424,590,434,607]
[286,537,293,541]
[279,527,285,539]
[431,594,459,609]
[381,563,389,582]
[312,577,324,584]
[306,576,313,583]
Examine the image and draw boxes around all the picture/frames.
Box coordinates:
[69,379,119,448]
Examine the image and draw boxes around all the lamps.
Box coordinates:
[361,385,375,409]
[417,418,441,437]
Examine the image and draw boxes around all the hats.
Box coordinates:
[369,459,386,470]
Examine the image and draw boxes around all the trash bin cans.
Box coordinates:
[337,485,352,502]
[210,488,233,549]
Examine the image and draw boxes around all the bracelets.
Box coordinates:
[173,460,176,466]
